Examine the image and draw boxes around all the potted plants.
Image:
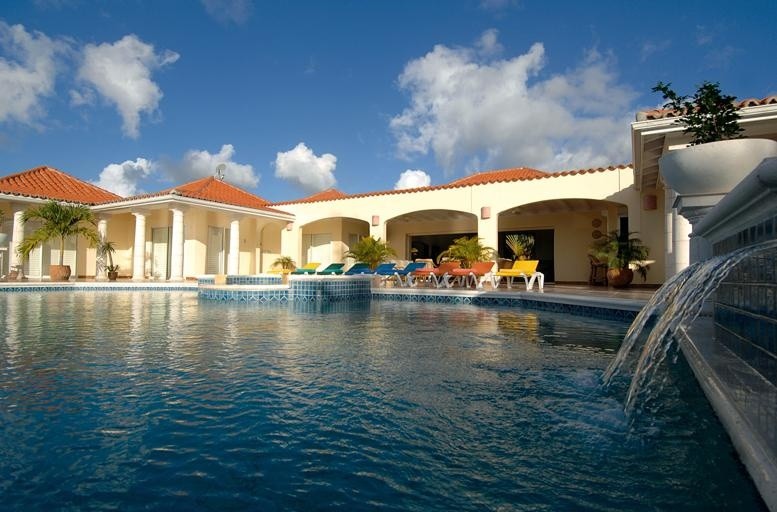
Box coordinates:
[103,242,120,281]
[588,229,650,286]
[649,77,777,195]
[14,199,101,281]
[272,256,296,274]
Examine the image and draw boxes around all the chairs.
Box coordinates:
[587,254,608,287]
[288,259,547,293]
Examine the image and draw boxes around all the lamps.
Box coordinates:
[285,222,292,231]
[642,195,657,211]
[371,215,379,226]
[480,206,490,219]
